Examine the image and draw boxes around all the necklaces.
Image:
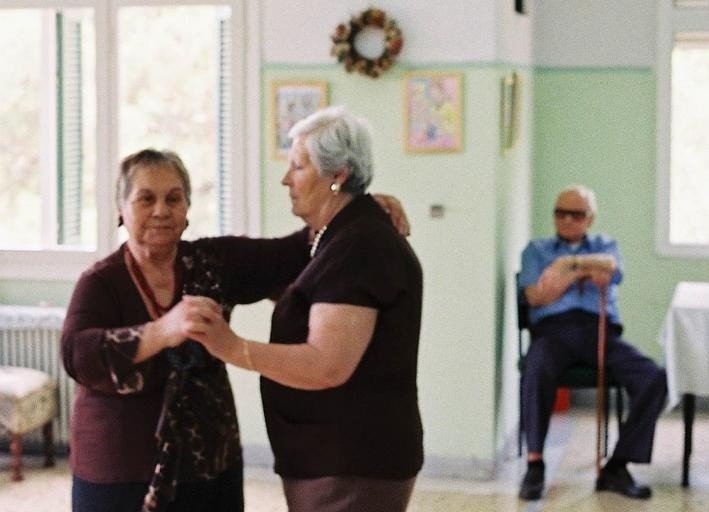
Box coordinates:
[309,225,327,258]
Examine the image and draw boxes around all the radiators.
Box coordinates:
[0,304,75,443]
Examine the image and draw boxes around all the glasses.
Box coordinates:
[553,207,592,222]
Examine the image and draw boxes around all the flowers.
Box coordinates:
[329,7,403,79]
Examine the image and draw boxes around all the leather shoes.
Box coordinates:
[516,460,547,502]
[592,463,656,498]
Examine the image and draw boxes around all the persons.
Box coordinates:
[181,104,423,511]
[60,148,409,511]
[518,185,667,500]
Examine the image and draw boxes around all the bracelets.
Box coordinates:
[243,338,256,371]
[571,252,579,270]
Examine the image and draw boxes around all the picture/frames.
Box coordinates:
[501,73,522,158]
[269,78,331,159]
[401,71,465,154]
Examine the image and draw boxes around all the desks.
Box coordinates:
[657,280,709,487]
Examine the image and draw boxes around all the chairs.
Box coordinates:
[515,271,623,458]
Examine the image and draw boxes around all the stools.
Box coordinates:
[0,364,58,482]
